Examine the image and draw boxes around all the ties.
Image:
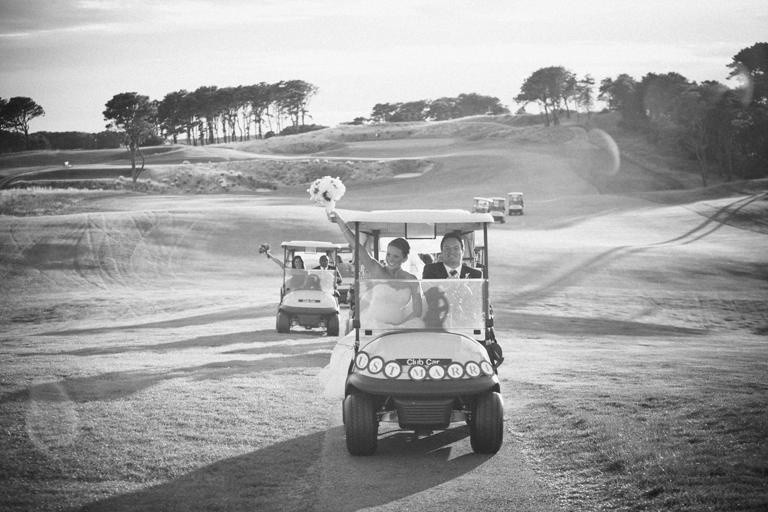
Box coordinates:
[450,271,458,276]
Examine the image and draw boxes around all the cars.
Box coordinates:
[472,191,524,225]
[336,207,504,458]
[333,242,358,305]
[275,240,340,337]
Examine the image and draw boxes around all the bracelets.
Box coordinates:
[342,228,348,233]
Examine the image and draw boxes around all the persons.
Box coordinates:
[420,232,482,332]
[320,209,424,403]
[266,253,310,289]
[336,256,343,263]
[312,255,341,298]
